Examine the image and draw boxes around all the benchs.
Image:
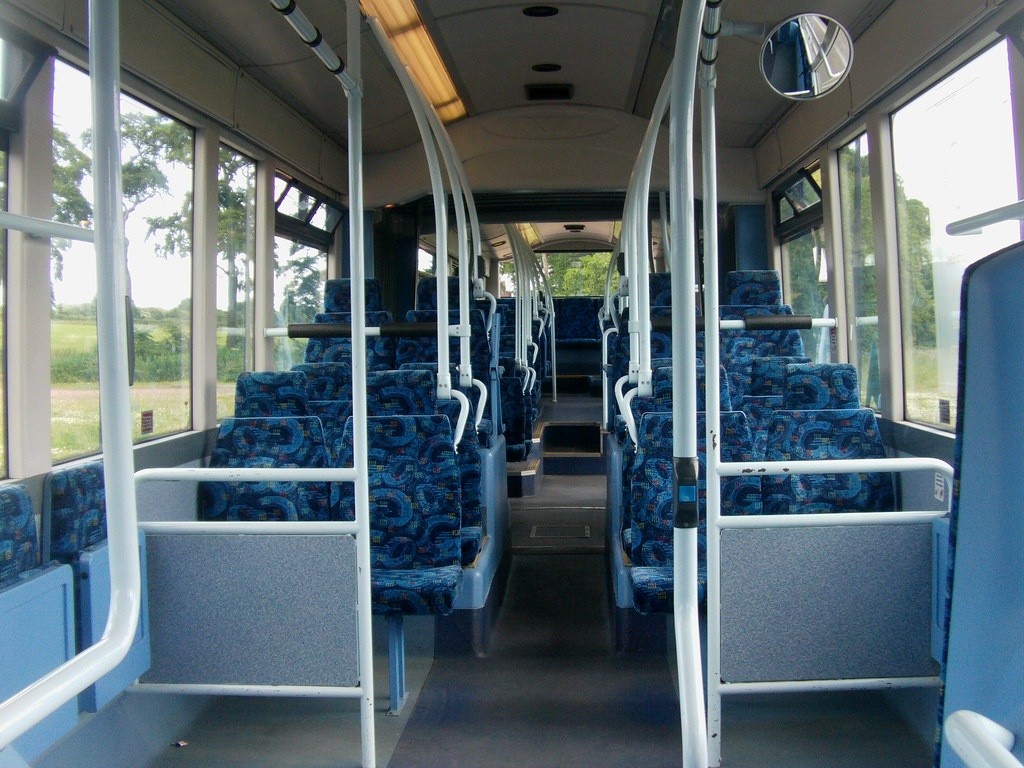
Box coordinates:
[199,268,898,714]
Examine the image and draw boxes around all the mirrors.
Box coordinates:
[758,10,852,105]
